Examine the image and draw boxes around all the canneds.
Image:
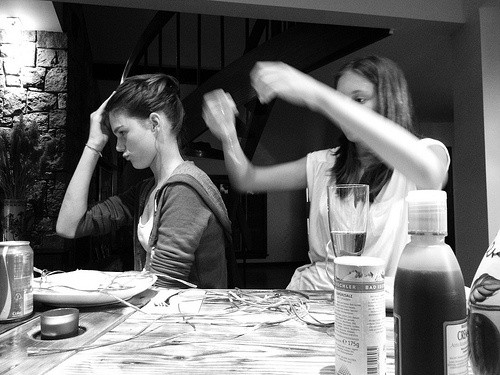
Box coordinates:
[0,240,33,323]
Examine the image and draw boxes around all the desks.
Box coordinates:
[0,288,473,375]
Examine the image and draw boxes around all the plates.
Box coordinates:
[27,269,160,307]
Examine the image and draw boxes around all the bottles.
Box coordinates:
[392,190,468,372]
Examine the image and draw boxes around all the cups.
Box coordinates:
[326,183,370,256]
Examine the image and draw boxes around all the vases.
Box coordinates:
[2,198,27,241]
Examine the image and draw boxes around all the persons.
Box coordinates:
[201,55,450,292]
[56,72,232,289]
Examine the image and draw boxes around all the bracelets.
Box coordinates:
[84,143,104,157]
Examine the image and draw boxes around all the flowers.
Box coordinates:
[0,112,45,198]
